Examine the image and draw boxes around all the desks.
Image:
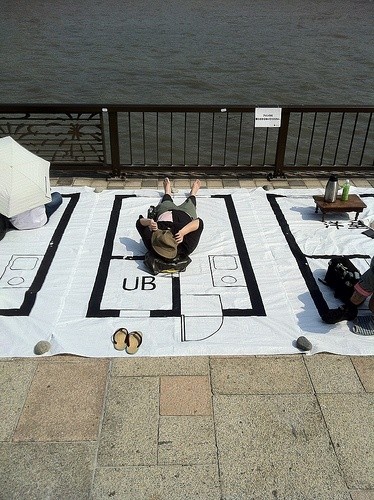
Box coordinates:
[312,194,367,222]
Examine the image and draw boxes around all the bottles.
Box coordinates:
[341,180,349,201]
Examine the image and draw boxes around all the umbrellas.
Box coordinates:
[0,136,52,218]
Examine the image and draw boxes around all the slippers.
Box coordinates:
[113,328,128,351]
[126,331,143,355]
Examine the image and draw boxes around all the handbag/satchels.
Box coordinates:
[325,256,362,302]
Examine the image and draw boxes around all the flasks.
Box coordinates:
[324,175,338,203]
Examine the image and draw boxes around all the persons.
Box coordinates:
[136,177,204,263]
[5,192,62,230]
[350,256,374,314]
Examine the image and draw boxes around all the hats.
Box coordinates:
[151,228,178,259]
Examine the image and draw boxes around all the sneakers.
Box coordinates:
[322,305,356,324]
